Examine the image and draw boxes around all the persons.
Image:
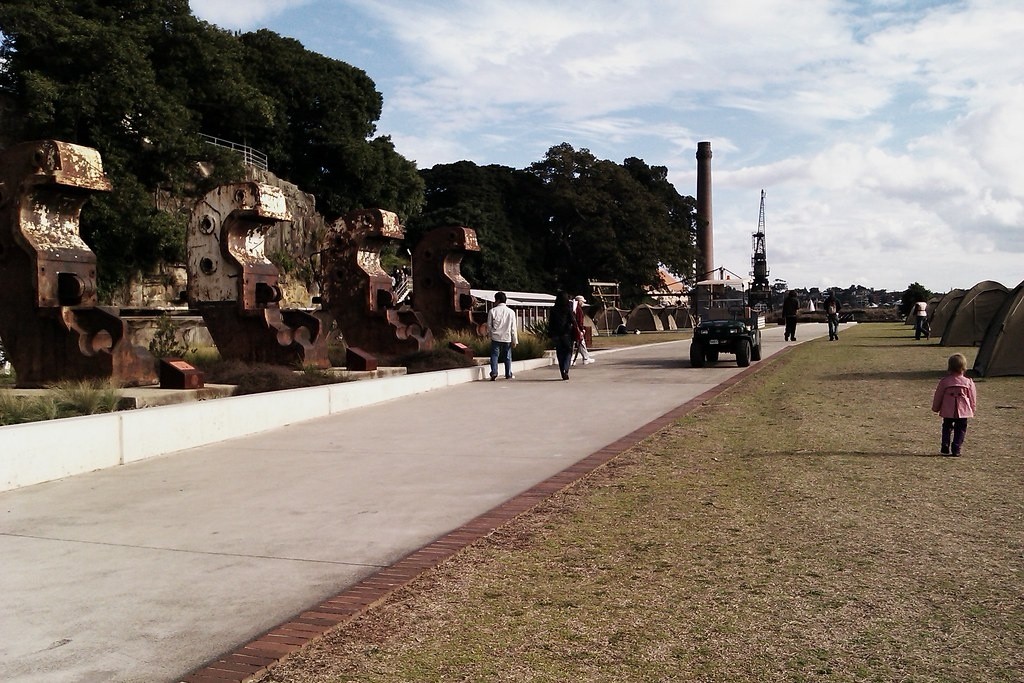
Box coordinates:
[487,291,519,380]
[781,289,800,341]
[931,353,977,457]
[911,295,931,340]
[823,290,842,341]
[571,296,595,365]
[545,289,583,380]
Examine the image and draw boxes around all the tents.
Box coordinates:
[973,280,1024,377]
[904,298,940,325]
[929,288,968,337]
[583,313,600,336]
[626,303,695,331]
[939,280,1010,348]
[593,306,626,330]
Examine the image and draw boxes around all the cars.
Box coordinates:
[690,279,762,366]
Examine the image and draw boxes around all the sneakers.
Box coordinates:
[571,360,576,366]
[583,358,595,365]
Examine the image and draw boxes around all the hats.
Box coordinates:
[575,295,586,302]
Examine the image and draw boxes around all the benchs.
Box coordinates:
[708,307,750,320]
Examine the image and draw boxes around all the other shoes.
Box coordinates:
[561,369,569,380]
[915,338,920,340]
[834,334,838,340]
[505,372,512,379]
[829,338,833,341]
[941,447,949,454]
[791,337,796,341]
[927,332,930,340]
[785,334,788,341]
[490,376,497,381]
[952,451,960,457]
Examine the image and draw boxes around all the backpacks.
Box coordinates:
[828,301,836,314]
